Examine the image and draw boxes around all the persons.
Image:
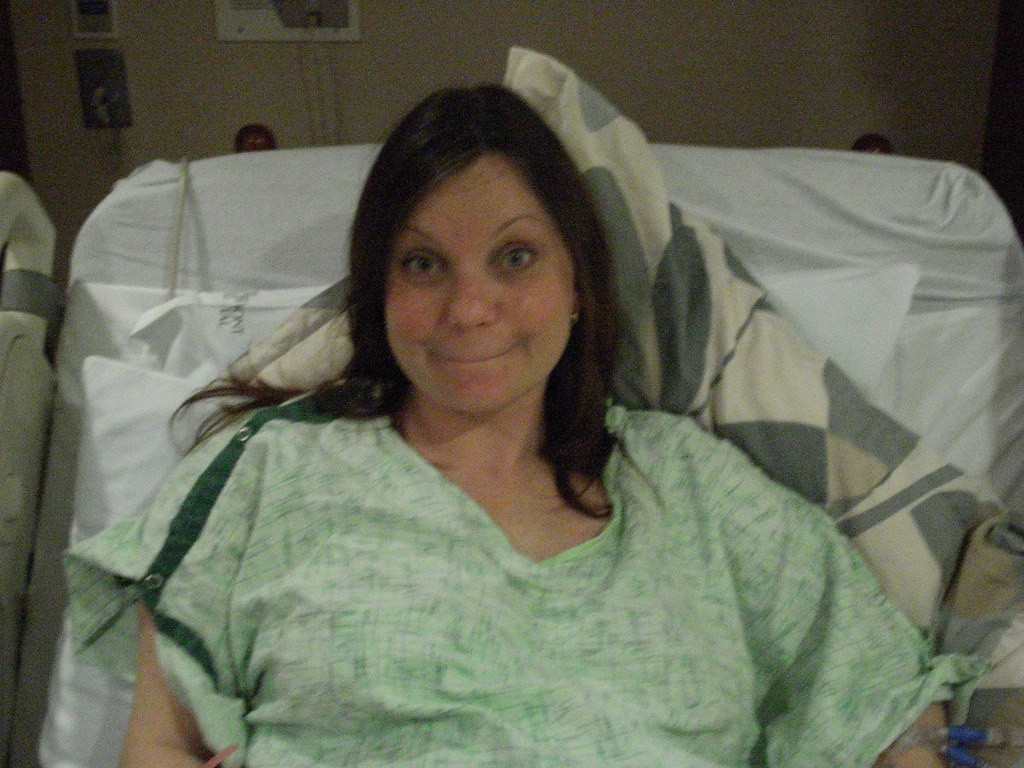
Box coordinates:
[61,84,991,768]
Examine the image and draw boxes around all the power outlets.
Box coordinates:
[73,47,133,127]
[215,0,361,43]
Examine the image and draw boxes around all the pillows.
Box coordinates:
[60,49,1024,768]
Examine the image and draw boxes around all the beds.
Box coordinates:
[5,140,1024,768]
